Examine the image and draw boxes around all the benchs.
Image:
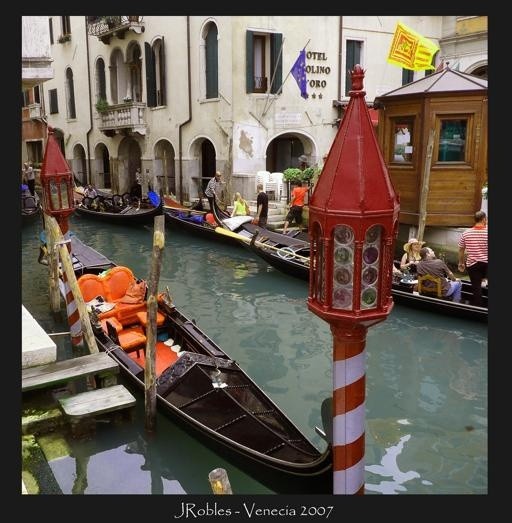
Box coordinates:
[77,266,146,333]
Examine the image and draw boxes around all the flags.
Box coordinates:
[291,50,309,100]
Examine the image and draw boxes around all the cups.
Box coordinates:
[403,275,408,282]
[410,275,415,282]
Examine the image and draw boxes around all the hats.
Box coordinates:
[403,238,426,253]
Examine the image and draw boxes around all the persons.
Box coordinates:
[204,171,227,212]
[229,191,249,218]
[457,211,489,306]
[156,175,164,198]
[75,181,100,209]
[282,181,310,235]
[135,168,142,198]
[254,184,268,227]
[399,238,427,292]
[22,163,35,203]
[417,247,462,303]
[146,168,154,192]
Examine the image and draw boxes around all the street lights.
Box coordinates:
[42,129,84,349]
[306,65,402,495]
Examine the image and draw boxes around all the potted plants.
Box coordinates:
[94,97,109,115]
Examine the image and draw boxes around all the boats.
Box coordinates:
[59,231,333,483]
[76,193,156,223]
[163,206,219,238]
[21,193,41,223]
[207,196,489,318]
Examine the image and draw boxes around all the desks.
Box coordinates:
[400,278,419,292]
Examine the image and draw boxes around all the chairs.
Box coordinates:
[106,320,146,359]
[254,171,288,201]
[418,274,447,300]
[136,302,168,337]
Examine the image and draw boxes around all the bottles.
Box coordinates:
[208,467,234,495]
